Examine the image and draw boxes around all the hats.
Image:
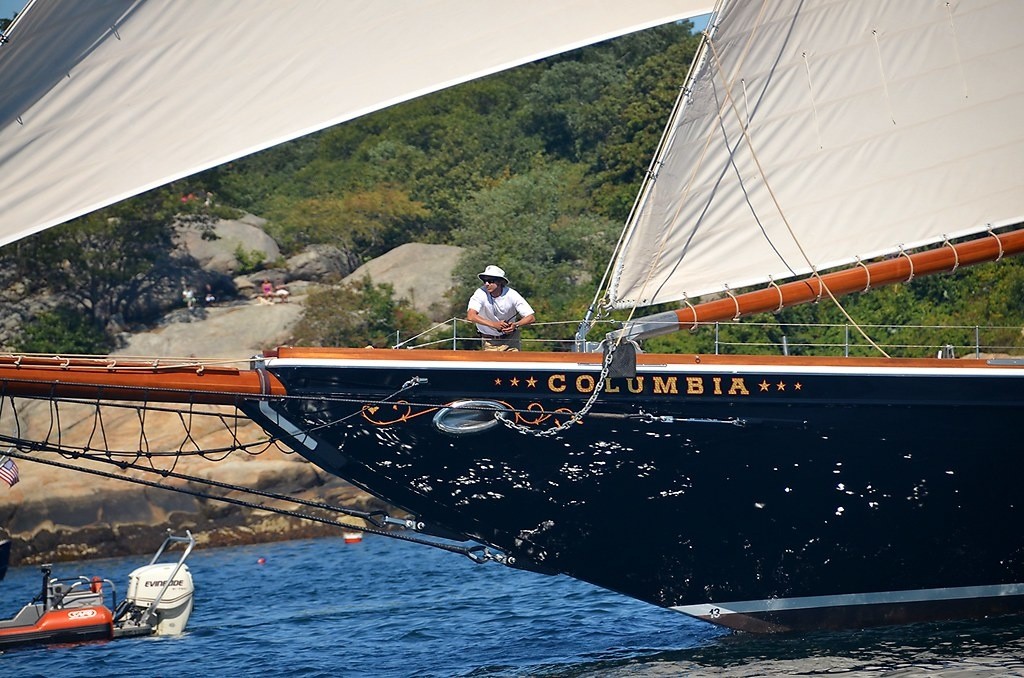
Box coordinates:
[477,265,509,286]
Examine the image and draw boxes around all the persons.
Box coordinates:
[262,278,275,305]
[204,284,215,306]
[182,189,214,206]
[467,266,536,352]
[182,284,196,311]
[276,278,290,303]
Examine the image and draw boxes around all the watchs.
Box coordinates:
[515,322,519,328]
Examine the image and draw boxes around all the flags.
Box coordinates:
[0,458,19,488]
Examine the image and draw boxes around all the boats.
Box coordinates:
[1,523,200,652]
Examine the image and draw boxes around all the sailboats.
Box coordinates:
[1,0,1023,630]
